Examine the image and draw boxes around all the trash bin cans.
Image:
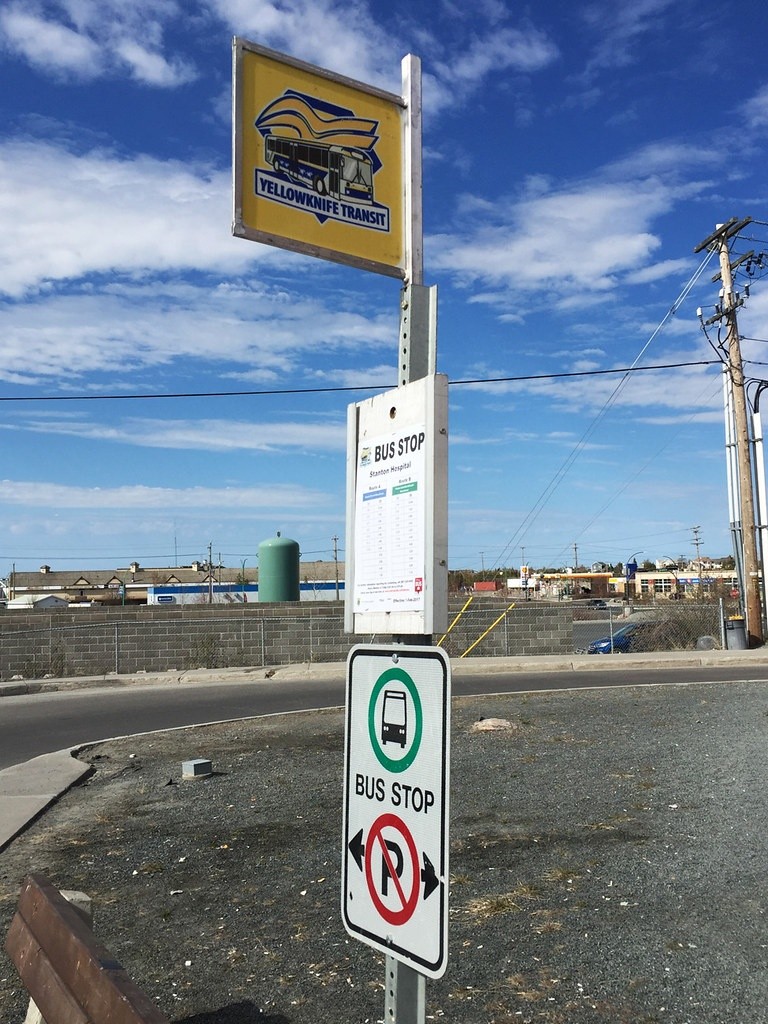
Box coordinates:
[624,607,633,617]
[724,619,746,649]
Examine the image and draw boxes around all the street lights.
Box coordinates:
[626,550,647,598]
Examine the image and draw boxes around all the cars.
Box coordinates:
[584,600,606,611]
[669,593,685,600]
[587,619,668,655]
